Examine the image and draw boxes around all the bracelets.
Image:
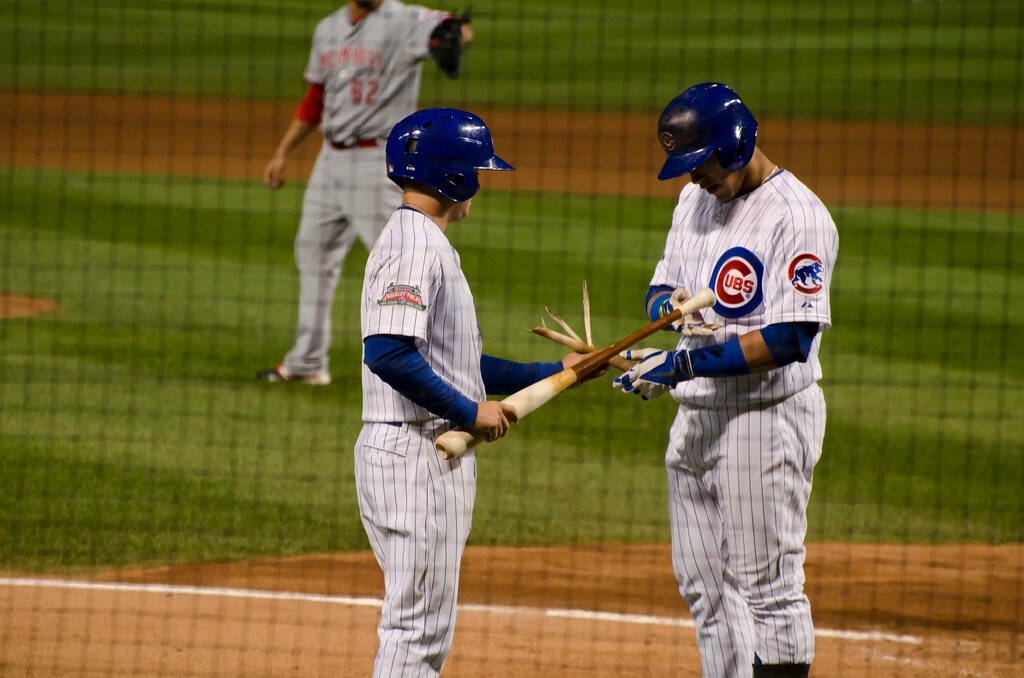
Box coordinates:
[659,299,668,317]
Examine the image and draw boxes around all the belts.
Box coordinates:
[329,136,377,151]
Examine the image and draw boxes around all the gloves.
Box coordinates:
[649,288,720,337]
[612,348,695,400]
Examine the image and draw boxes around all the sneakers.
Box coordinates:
[256,365,330,386]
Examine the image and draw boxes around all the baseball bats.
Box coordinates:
[433,289,717,452]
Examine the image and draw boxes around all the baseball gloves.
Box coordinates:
[430,11,467,78]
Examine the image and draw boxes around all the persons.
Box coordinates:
[258,0,473,385]
[612,81,839,677]
[355,108,612,678]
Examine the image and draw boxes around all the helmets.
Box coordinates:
[385,108,515,202]
[657,82,758,181]
